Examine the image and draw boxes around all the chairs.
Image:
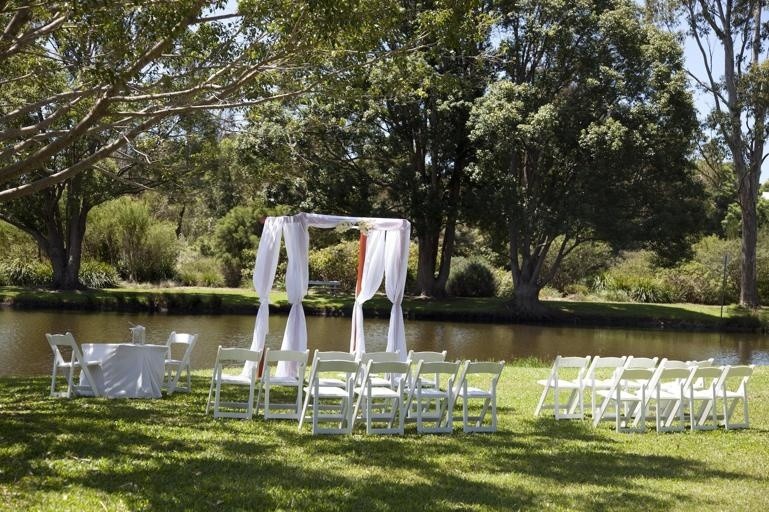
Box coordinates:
[164,331,199,396]
[129,325,145,344]
[45,332,99,399]
[534,355,756,435]
[205,345,506,437]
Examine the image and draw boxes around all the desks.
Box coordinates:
[81,343,168,399]
